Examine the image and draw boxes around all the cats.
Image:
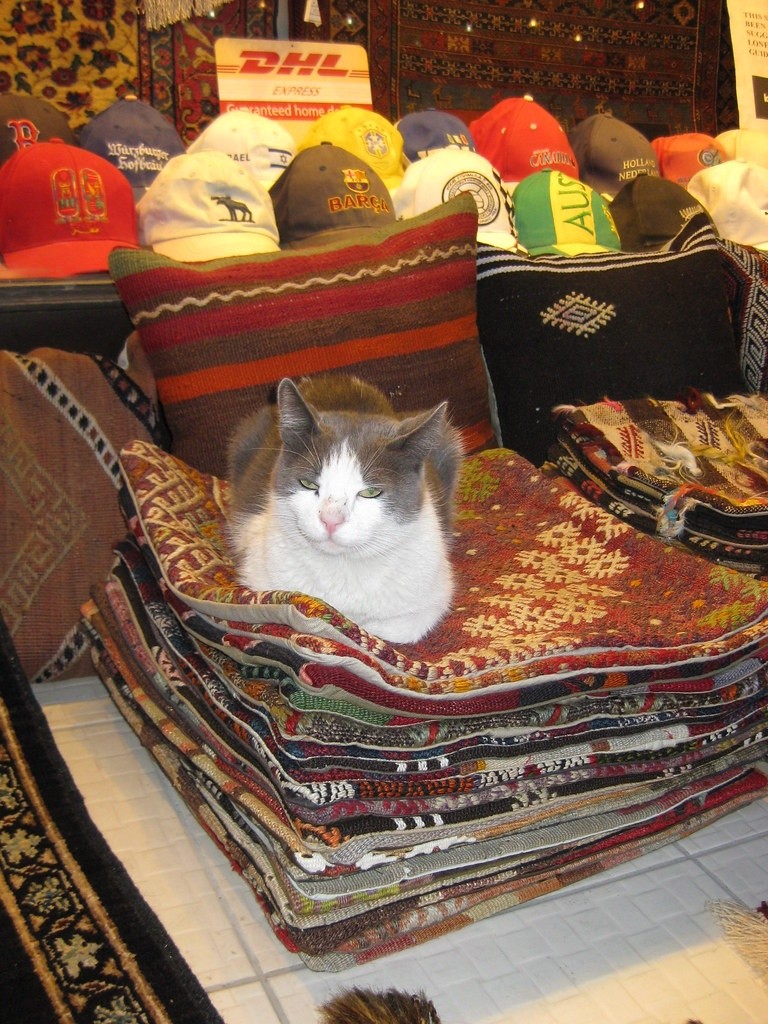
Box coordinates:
[225,372,464,645]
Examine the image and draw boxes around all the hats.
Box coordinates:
[132,153,282,265]
[0,93,76,170]
[79,94,186,204]
[185,110,299,193]
[395,93,768,280]
[0,139,140,279]
[296,107,406,191]
[269,141,397,249]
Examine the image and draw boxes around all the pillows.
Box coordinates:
[108,189,505,477]
